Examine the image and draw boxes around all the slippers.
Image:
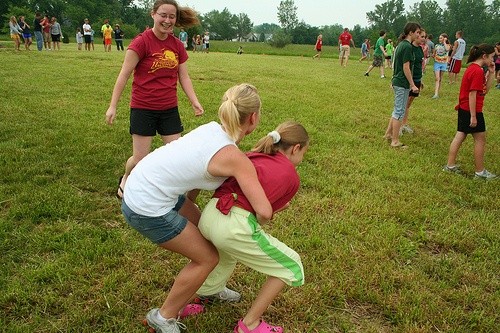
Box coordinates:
[116,175,123,199]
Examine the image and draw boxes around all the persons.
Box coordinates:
[384,22,425,147]
[9,15,32,52]
[105,0,204,199]
[170,27,210,53]
[358,30,395,78]
[443,43,499,180]
[237,46,245,55]
[338,28,355,66]
[76,18,94,51]
[421,31,466,99]
[121,83,309,333]
[101,19,125,52]
[482,42,500,89]
[313,35,322,61]
[33,12,63,51]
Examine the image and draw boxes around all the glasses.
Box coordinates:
[156,12,176,19]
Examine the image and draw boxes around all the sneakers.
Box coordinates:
[474,169,498,180]
[399,124,414,135]
[442,165,468,176]
[142,308,181,333]
[212,287,241,302]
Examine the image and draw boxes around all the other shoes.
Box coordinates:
[432,94,439,99]
[391,142,409,148]
[449,81,454,86]
[364,72,369,76]
[381,75,387,78]
[15,48,22,52]
[24,48,30,51]
[384,135,393,141]
[44,48,52,50]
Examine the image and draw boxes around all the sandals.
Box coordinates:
[234,317,283,333]
[178,297,207,317]
